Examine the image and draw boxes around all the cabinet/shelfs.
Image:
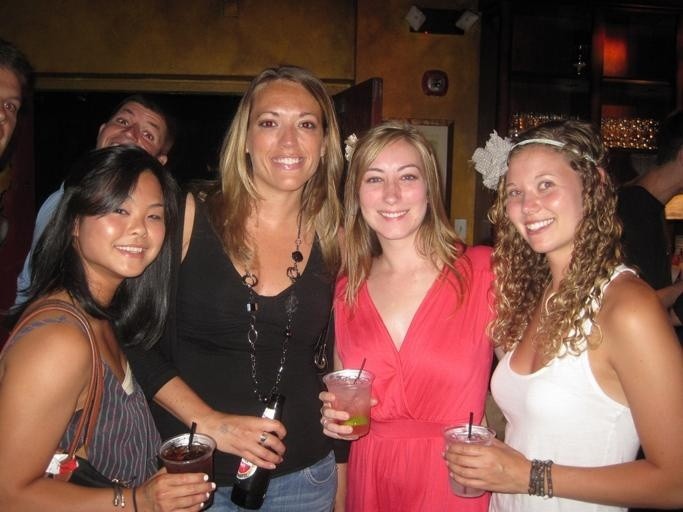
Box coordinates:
[470,0,682,257]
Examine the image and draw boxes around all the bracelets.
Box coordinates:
[130,483,139,511]
[527,457,554,499]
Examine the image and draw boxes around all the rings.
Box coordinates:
[322,418,328,426]
[258,432,268,443]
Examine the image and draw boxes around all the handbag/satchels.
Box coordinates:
[0,300,124,488]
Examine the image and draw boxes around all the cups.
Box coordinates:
[321,368,375,439]
[443,426,497,499]
[157,432,217,511]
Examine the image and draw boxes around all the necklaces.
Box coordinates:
[244,215,302,404]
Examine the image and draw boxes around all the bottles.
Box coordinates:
[231,394,286,510]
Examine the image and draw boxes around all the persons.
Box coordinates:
[441,118,681,512]
[10,96,177,316]
[0,40,36,243]
[317,119,506,512]
[120,66,350,511]
[1,143,217,511]
[611,119,681,329]
